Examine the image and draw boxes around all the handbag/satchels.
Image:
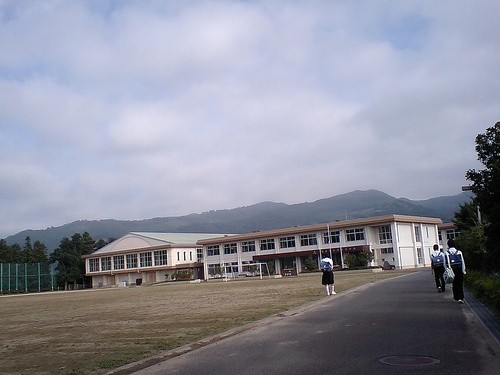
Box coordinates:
[443,268,455,283]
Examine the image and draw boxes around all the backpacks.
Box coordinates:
[447,250,461,266]
[432,252,444,267]
[322,259,331,272]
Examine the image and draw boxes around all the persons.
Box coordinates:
[430,244,448,294]
[320,258,337,295]
[446,239,466,304]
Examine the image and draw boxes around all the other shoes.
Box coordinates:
[453,299,458,303]
[437,287,441,292]
[326,292,330,295]
[458,300,464,304]
[331,292,336,295]
